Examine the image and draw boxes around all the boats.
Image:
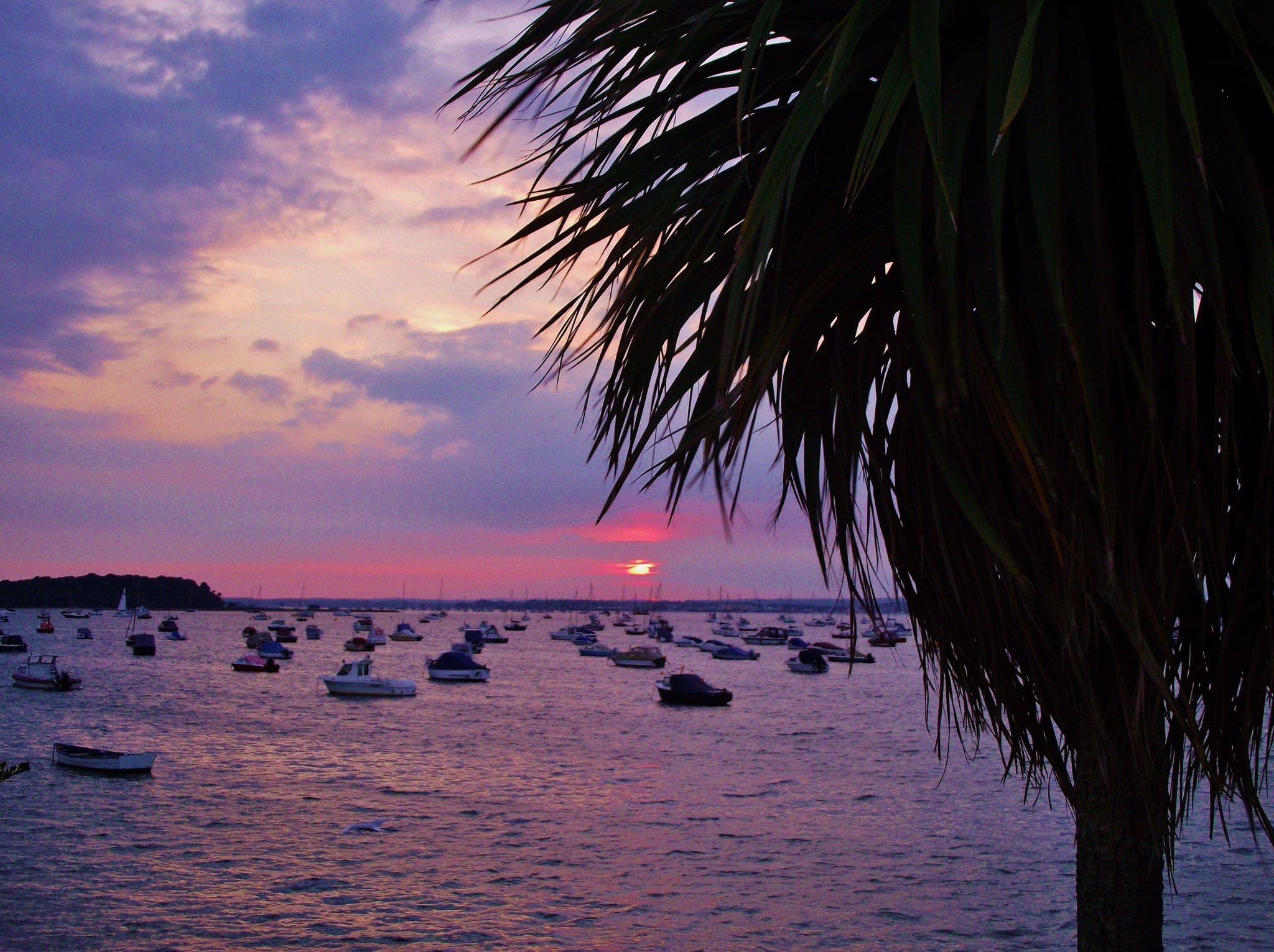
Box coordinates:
[579,641,620,657]
[77,627,94,639]
[611,644,666,669]
[251,613,271,621]
[675,635,703,647]
[343,636,374,652]
[133,633,156,655]
[162,602,179,620]
[387,630,424,641]
[786,650,828,673]
[52,742,157,774]
[306,625,322,639]
[297,617,307,621]
[655,672,734,705]
[11,652,81,689]
[697,639,733,651]
[157,618,188,641]
[711,646,760,660]
[367,627,386,644]
[786,638,809,649]
[869,632,907,647]
[741,627,787,644]
[0,632,27,651]
[827,648,876,663]
[319,657,417,697]
[231,619,298,672]
[808,641,843,653]
[292,582,315,617]
[425,620,510,681]
[1,608,16,623]
[335,608,373,630]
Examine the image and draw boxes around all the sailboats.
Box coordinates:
[419,578,448,623]
[246,585,266,613]
[457,581,674,646]
[59,589,102,619]
[396,581,415,634]
[34,588,54,632]
[705,584,912,638]
[186,585,197,612]
[114,581,153,646]
